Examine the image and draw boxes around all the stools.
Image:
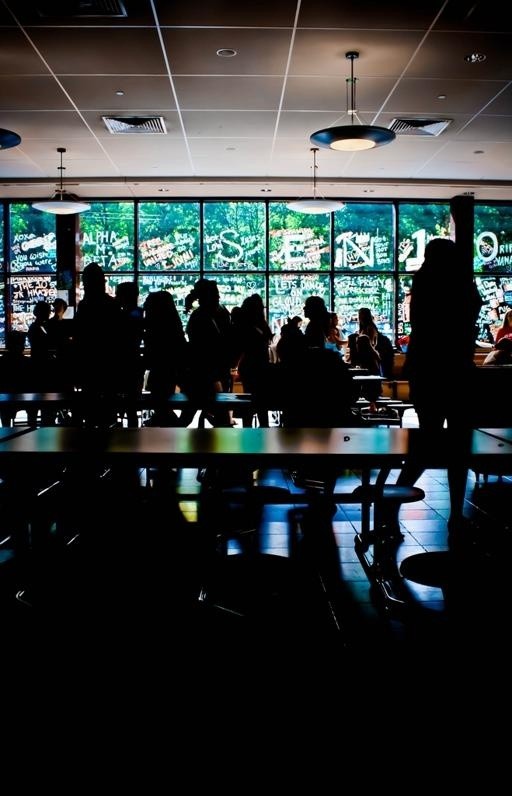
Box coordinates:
[0,428,512,618]
[351,484,424,616]
[350,396,414,428]
[402,554,451,586]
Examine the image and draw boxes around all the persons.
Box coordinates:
[0,240,511,542]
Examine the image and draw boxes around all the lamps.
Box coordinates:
[32,147,91,215]
[310,51,396,152]
[286,148,345,215]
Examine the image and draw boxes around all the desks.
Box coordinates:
[0,427,31,440]
[1,392,252,408]
[349,368,369,375]
[478,428,512,445]
[352,374,389,395]
